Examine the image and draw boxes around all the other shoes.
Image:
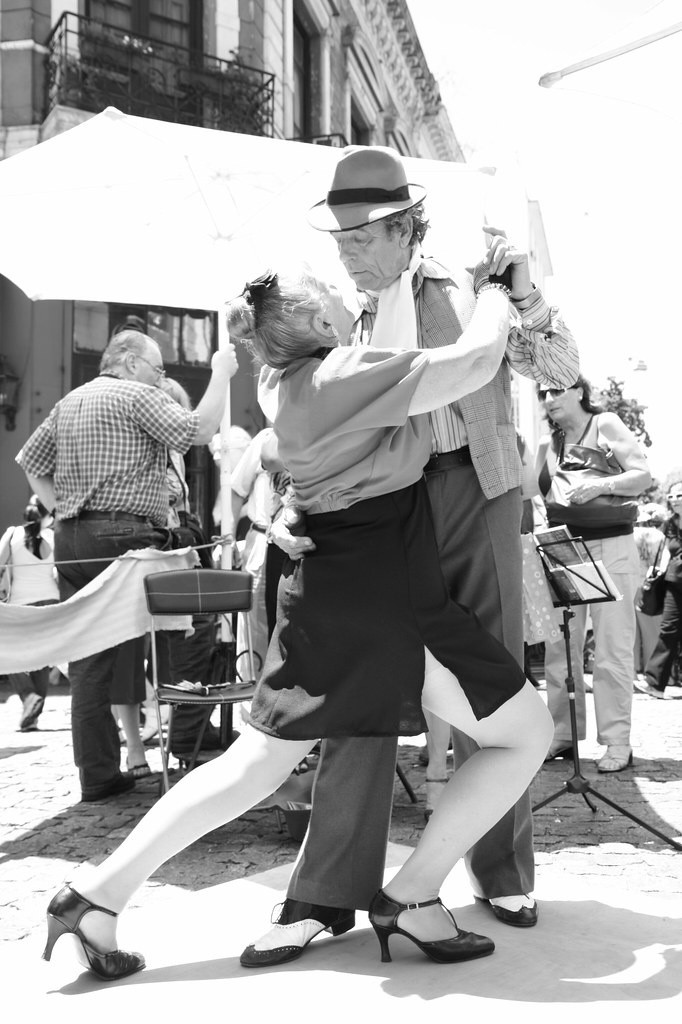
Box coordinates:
[24,722,39,731]
[82,771,134,802]
[597,748,634,772]
[636,681,664,698]
[21,692,43,728]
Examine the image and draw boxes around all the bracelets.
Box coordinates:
[51,506,56,516]
[474,282,513,299]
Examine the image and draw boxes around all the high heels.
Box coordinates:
[545,745,573,762]
[367,888,494,963]
[41,882,147,981]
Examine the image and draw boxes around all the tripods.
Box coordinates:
[532,535,682,850]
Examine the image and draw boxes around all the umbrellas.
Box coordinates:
[0,107,532,645]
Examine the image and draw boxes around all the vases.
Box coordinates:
[80,40,150,74]
[175,69,233,97]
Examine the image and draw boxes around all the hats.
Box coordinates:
[305,146,428,232]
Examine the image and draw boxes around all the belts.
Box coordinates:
[75,511,148,523]
[250,524,265,533]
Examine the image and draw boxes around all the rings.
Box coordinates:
[580,494,583,499]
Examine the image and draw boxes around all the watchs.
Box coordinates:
[608,477,616,496]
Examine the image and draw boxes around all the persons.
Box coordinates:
[0,147,682,979]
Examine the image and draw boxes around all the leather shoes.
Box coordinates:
[241,899,355,969]
[474,890,538,926]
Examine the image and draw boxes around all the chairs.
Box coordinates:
[144,568,258,798]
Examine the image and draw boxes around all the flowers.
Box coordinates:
[79,21,154,55]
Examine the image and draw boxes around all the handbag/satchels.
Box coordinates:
[640,572,667,615]
[545,443,639,526]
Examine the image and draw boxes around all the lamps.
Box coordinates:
[0,354,21,431]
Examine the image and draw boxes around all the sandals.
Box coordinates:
[126,756,153,777]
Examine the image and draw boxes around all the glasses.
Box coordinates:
[139,356,166,379]
[667,493,682,500]
[538,386,573,400]
